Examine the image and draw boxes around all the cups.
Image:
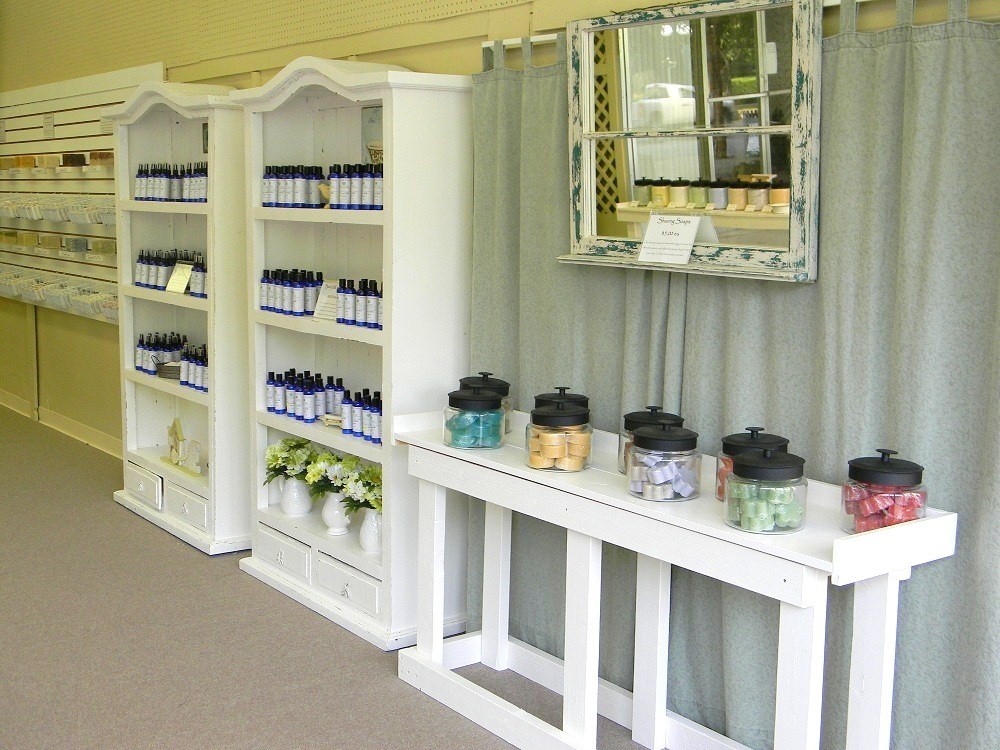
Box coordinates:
[633,176,789,210]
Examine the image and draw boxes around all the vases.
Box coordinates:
[359,506,383,553]
[281,475,312,515]
[322,491,350,536]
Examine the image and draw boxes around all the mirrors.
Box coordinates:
[556,2,821,283]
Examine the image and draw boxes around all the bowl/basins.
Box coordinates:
[770,203,790,214]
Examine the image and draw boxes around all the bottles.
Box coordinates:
[619,406,701,501]
[132,161,384,445]
[440,373,509,451]
[839,450,928,534]
[524,387,594,472]
[716,427,806,534]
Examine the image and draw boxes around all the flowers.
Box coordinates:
[341,468,382,516]
[305,450,359,499]
[262,436,315,484]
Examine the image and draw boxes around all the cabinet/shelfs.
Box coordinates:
[228,53,472,651]
[98,80,249,556]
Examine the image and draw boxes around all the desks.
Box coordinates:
[393,410,958,750]
